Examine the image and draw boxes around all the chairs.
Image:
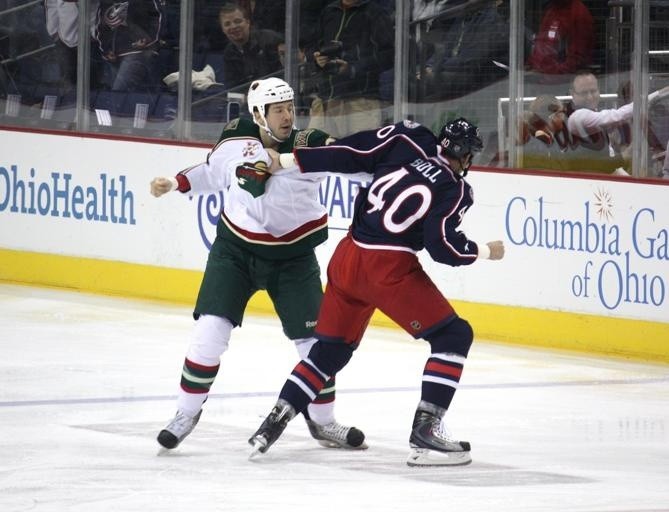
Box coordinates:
[0,0,668,176]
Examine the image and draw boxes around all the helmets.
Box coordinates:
[439,118,483,160]
[246,77,296,130]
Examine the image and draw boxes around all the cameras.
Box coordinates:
[319,39,344,74]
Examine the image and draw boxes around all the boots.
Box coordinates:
[249,401,294,451]
[408,410,470,451]
[157,397,209,449]
[305,415,365,448]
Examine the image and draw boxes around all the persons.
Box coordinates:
[247,115,507,455]
[1,0,509,127]
[519,1,598,87]
[148,77,368,450]
[481,71,667,181]
